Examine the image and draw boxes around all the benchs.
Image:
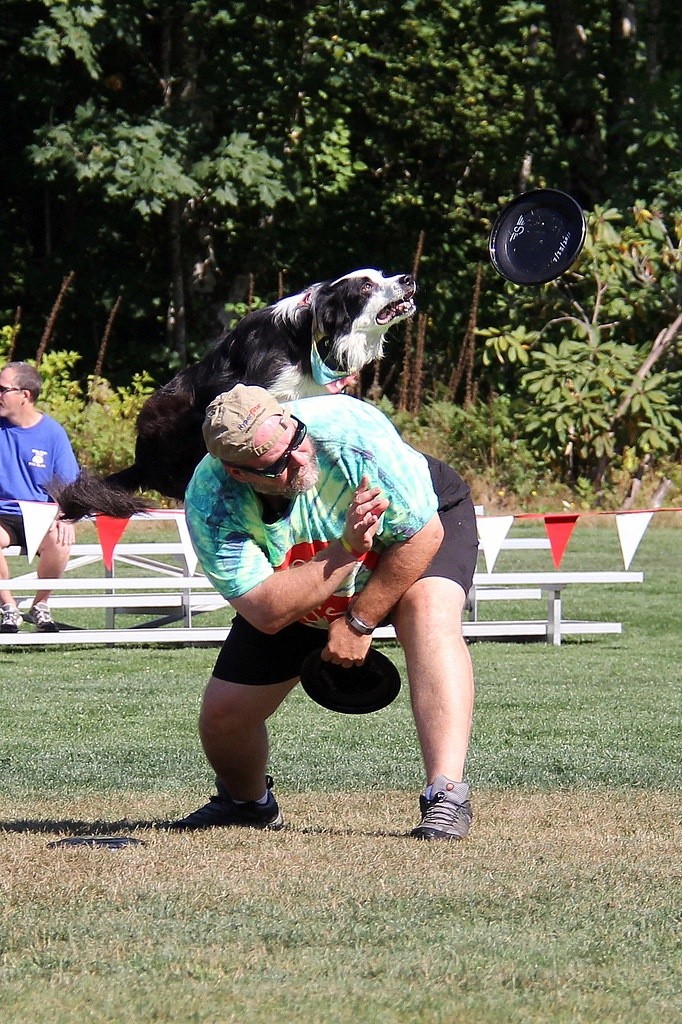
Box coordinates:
[0,509,645,647]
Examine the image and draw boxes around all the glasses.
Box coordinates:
[239,415,307,477]
[1,387,21,393]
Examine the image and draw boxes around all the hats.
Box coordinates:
[202,383,291,462]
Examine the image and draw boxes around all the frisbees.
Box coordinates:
[299,642,403,715]
[490,187,587,288]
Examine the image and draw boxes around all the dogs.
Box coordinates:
[53,267,419,528]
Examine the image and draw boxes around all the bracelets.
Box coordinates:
[346,608,374,636]
[339,533,368,561]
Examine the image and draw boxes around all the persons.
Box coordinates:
[182,381,478,836]
[0,363,81,632]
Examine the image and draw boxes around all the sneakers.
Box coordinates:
[0,603,25,632]
[25,603,60,632]
[410,775,472,840]
[169,775,284,831]
